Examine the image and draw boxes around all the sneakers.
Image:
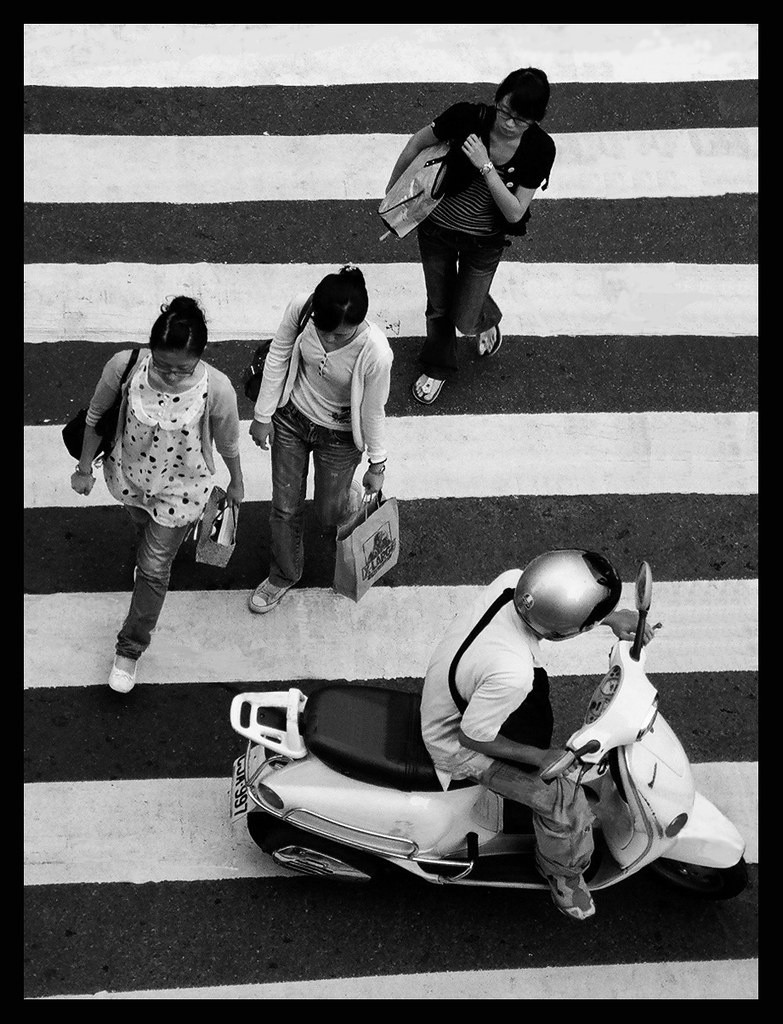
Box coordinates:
[248,573,304,613]
[538,855,596,920]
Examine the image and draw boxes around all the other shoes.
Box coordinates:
[108,653,138,693]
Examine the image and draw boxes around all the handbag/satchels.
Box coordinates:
[377,101,488,239]
[334,489,401,604]
[488,667,555,775]
[195,486,242,567]
[61,346,140,462]
[245,293,315,403]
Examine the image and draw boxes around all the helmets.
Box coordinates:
[514,548,622,643]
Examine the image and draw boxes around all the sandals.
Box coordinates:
[413,373,446,405]
[475,325,502,359]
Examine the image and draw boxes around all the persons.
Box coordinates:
[71,297,245,694]
[249,264,394,614]
[420,549,655,920]
[386,65,556,403]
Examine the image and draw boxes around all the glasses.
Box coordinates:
[152,358,199,378]
[495,104,531,128]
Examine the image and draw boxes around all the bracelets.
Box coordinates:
[75,464,93,476]
[368,464,386,475]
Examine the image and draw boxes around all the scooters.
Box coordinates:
[230,557,750,904]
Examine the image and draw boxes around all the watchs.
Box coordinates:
[480,162,494,176]
